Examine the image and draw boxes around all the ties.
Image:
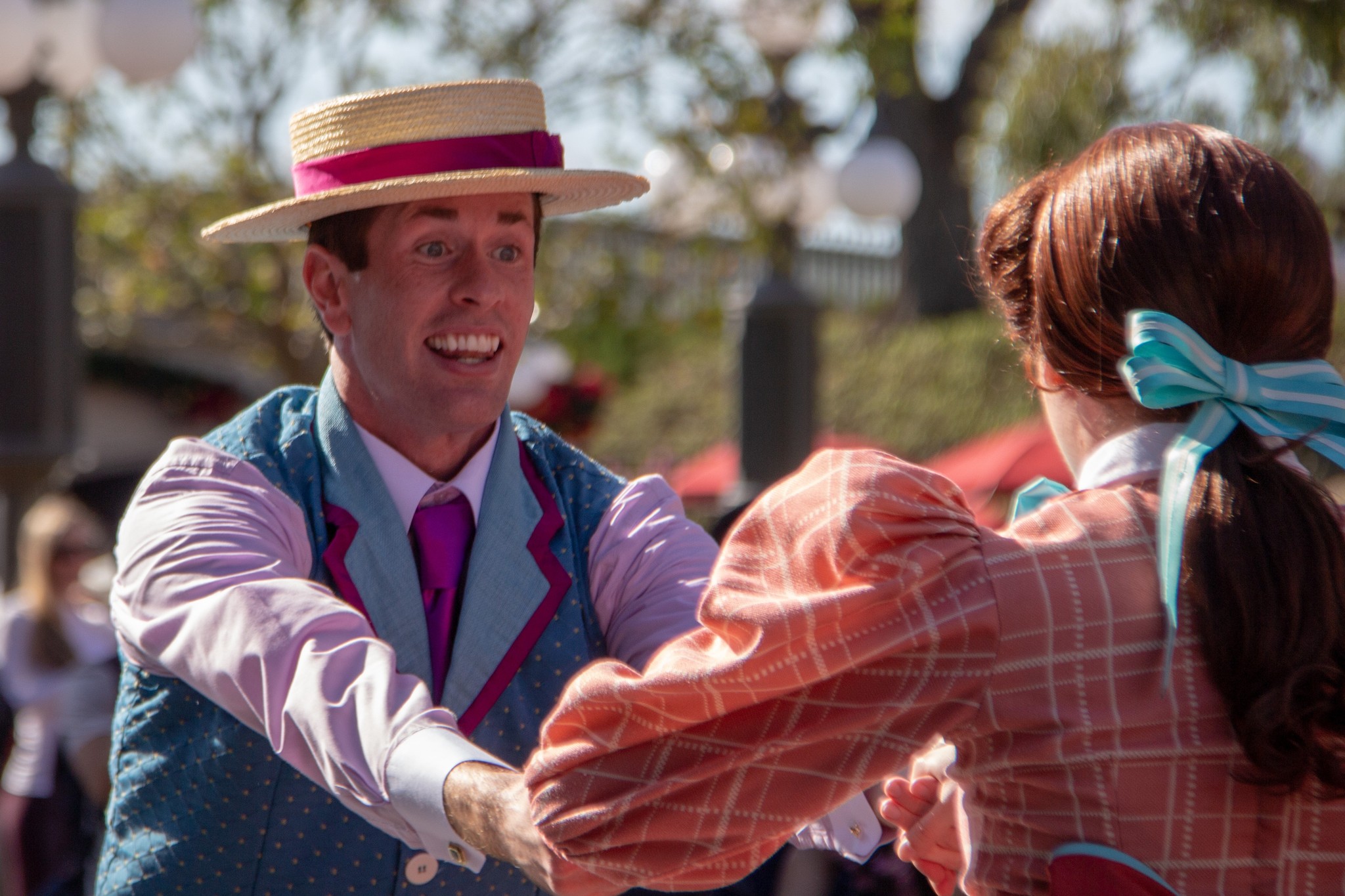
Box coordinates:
[414,491,475,700]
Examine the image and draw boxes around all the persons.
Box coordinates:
[95,81,968,896]
[525,123,1345,896]
[0,495,122,896]
[507,347,610,447]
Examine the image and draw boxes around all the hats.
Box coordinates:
[200,79,649,243]
